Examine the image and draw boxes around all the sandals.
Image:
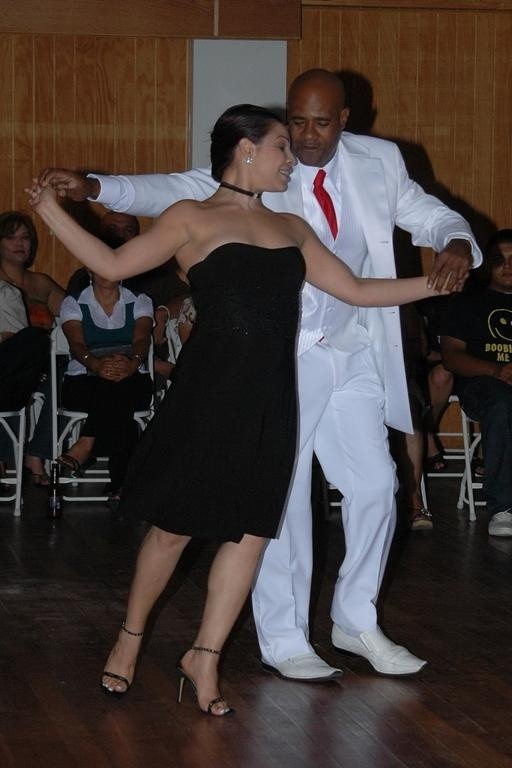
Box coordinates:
[99,625,144,698]
[105,496,120,508]
[55,454,79,471]
[407,505,433,530]
[32,473,51,487]
[423,452,445,470]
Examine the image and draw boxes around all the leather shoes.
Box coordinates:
[331,623,429,678]
[257,652,343,683]
[487,512,512,537]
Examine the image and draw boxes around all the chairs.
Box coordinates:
[0,410,32,519]
[48,322,156,518]
[326,398,486,528]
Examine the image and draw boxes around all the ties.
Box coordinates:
[313,170,337,240]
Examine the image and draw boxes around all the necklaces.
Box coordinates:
[218,180,262,201]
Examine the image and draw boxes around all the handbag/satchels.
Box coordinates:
[87,344,133,377]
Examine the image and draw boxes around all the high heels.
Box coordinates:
[177,646,231,717]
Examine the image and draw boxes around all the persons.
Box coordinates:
[21,101,472,720]
[38,67,486,682]
[1,208,511,537]
